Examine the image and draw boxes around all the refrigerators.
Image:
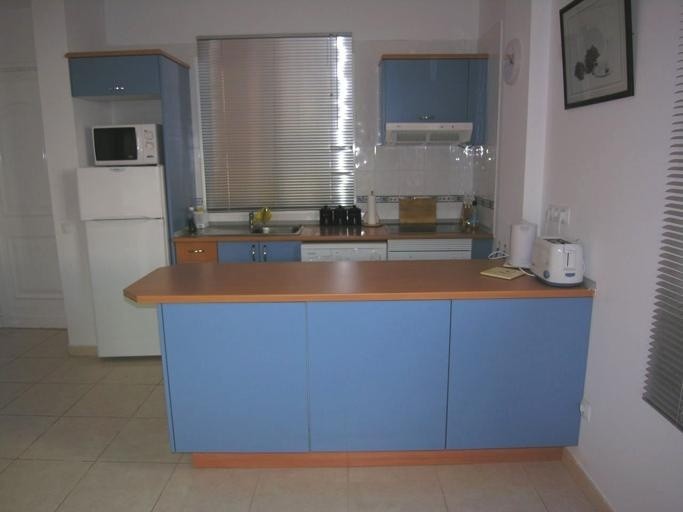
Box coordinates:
[77,166,170,357]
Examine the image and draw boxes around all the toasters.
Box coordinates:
[532,236,583,288]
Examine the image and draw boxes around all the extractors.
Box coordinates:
[387,125,464,145]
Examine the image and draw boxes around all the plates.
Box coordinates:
[362,223,383,227]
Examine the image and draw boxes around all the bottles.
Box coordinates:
[320,205,361,225]
[186,207,196,234]
[368,191,376,225]
[471,200,478,227]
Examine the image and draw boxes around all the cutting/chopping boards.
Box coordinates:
[400,198,435,224]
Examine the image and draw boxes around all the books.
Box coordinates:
[478,266,526,280]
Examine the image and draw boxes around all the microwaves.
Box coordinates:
[91,123,164,165]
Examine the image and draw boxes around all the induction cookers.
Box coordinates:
[386,222,463,233]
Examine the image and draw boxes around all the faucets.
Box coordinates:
[249,212,263,232]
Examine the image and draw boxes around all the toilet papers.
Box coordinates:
[368,195,376,224]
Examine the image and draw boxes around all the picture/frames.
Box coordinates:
[559,0,633,109]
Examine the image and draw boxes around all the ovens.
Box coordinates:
[388,239,472,260]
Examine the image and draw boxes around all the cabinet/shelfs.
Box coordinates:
[175,242,217,265]
[218,241,301,263]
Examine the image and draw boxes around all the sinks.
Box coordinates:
[256,224,304,236]
